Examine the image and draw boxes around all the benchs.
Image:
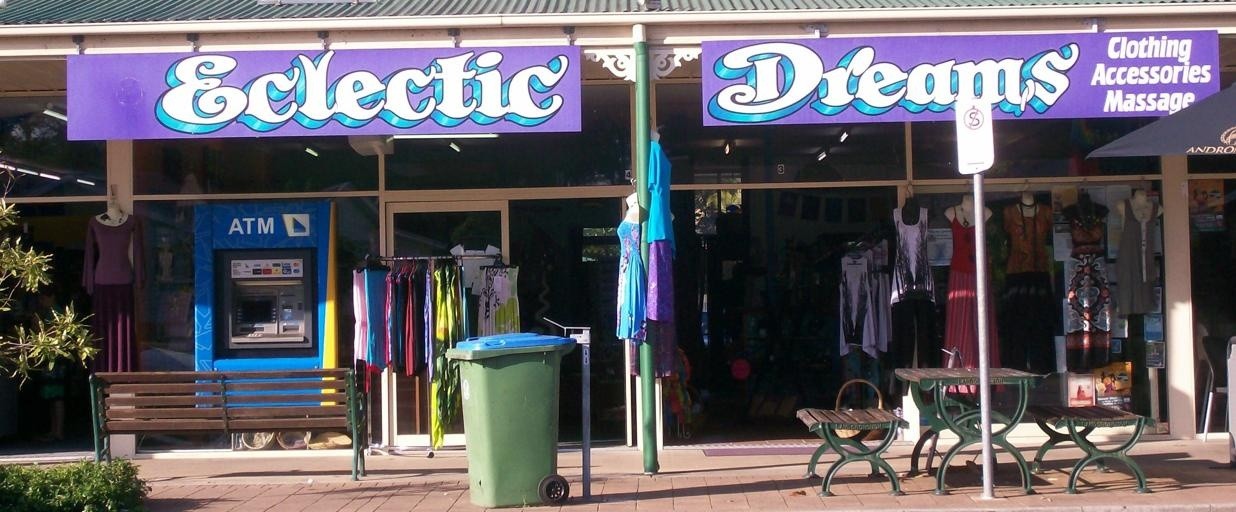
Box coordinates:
[1021,403,1152,496]
[797,406,910,498]
[86,362,367,484]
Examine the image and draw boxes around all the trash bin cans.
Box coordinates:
[445,332,576,508]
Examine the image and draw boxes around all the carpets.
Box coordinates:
[701,445,889,457]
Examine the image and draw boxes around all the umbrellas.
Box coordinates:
[1084,81,1236,159]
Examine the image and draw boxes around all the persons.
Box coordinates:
[614,194,644,346]
[1002,193,1053,371]
[1065,193,1113,355]
[646,131,675,323]
[942,192,1007,396]
[83,206,145,374]
[887,194,938,311]
[1113,187,1166,318]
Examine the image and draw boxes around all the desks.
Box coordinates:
[893,366,1048,497]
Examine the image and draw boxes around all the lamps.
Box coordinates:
[814,129,851,163]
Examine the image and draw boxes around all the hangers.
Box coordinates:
[844,232,885,258]
[355,251,518,279]
